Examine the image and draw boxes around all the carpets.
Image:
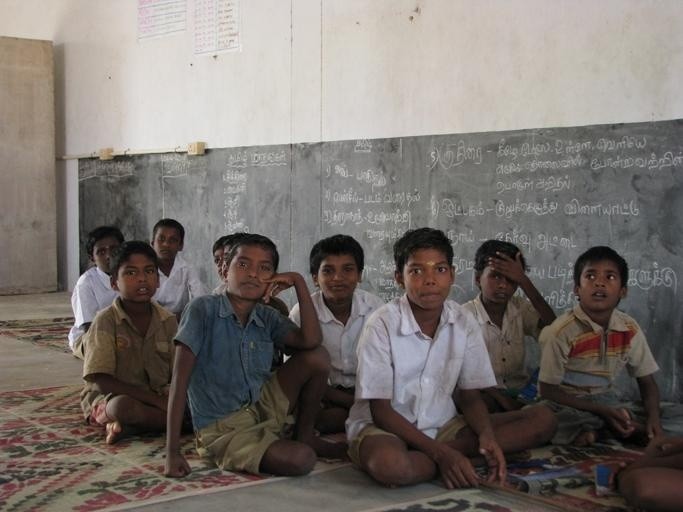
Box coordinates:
[0,382,647,511]
[0,313,88,358]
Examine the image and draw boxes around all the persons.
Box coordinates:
[521,245,663,453]
[80,240,178,443]
[453,238,558,413]
[617,438,683,512]
[164,234,350,479]
[69,226,125,359]
[152,218,205,324]
[283,233,387,434]
[210,232,290,317]
[345,226,558,491]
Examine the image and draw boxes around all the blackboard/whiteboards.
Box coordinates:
[78,118,683,406]
